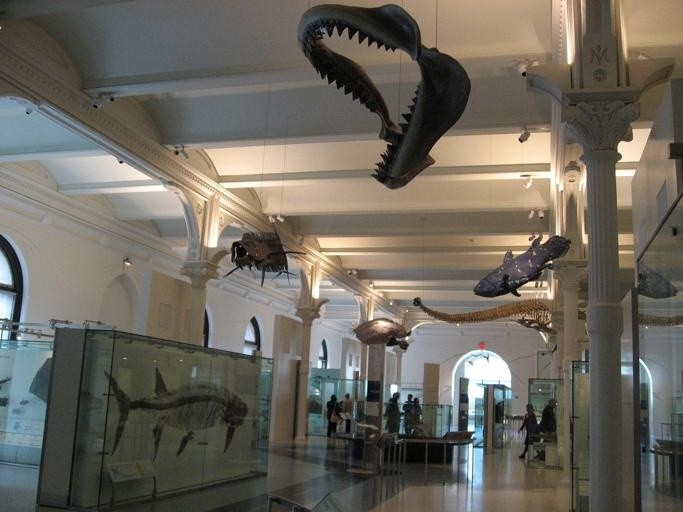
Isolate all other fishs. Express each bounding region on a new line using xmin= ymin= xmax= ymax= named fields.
xmin=103 ymin=367 xmax=248 ymax=464
xmin=356 ymin=423 xmax=379 ymax=432
xmin=473 ymin=234 xmax=571 ymax=297
xmin=356 ymin=318 xmax=409 ymax=346
xmin=637 ymin=259 xmax=678 ymax=299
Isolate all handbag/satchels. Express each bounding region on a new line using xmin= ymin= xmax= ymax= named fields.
xmin=331 ymin=413 xmax=338 ymax=422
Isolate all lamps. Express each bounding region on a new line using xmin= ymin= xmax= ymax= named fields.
xmin=120 ymin=257 xmax=132 ymax=270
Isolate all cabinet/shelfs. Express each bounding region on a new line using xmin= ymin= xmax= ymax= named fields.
xmin=474 ymin=386 xmax=513 ymax=448
xmin=536 ymin=350 xmax=553 ymax=378
xmin=308 ymin=368 xmax=340 ymax=432
xmin=383 ymin=383 xmax=424 ymax=403
xmin=0 ymin=338 xmax=55 ymax=466
xmin=525 ymin=378 xmax=565 ymax=470
xmin=321 ymin=378 xmax=365 ymax=435
xmin=634 ymin=193 xmax=683 ymax=512
xmin=569 ymin=360 xmax=589 ymax=511
xmin=35 ymin=329 xmax=275 ymax=512
xmin=349 ymin=400 xmax=454 ymax=439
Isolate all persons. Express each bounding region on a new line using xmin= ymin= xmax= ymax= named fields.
xmin=518 ymin=405 xmax=540 ymax=458
xmin=538 ymin=399 xmax=556 ymax=460
xmin=384 ymin=393 xmax=422 ymax=435
xmin=327 ymin=393 xmax=353 ymax=438
